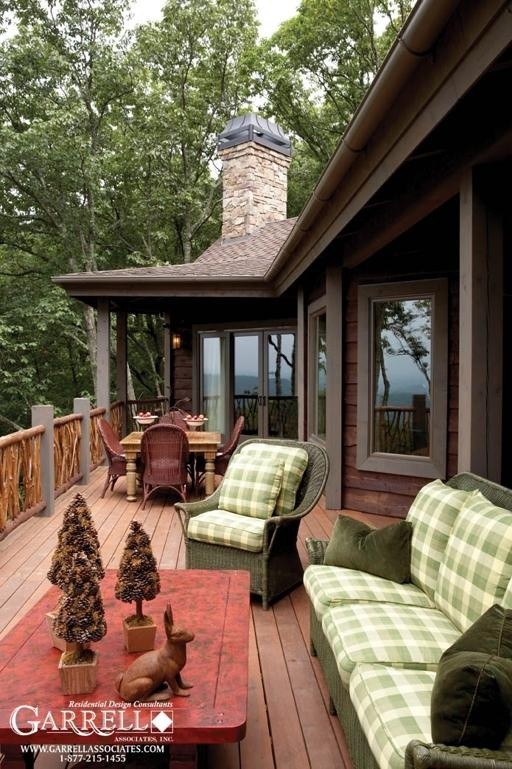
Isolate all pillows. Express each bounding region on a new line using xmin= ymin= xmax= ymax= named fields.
xmin=323 ymin=514 xmax=412 ymax=584
xmin=217 ymin=454 xmax=285 ymax=519
xmin=430 ymin=602 xmax=512 ymax=748
xmin=434 ymin=489 xmax=512 ymax=635
xmin=404 ymin=478 xmax=475 ymax=603
xmin=240 ymin=443 xmax=308 ymax=515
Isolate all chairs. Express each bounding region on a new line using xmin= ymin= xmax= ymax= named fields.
xmin=96 ymin=411 xmax=245 ymax=511
xmin=173 ymin=437 xmax=330 ymax=611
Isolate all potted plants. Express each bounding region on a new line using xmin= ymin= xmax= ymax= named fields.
xmin=45 ymin=491 xmax=160 ymax=696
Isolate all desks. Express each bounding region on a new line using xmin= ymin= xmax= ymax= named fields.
xmin=0 ymin=569 xmax=252 ymax=769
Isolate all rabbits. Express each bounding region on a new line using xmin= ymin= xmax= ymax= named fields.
xmin=113 ymin=600 xmax=196 ymax=702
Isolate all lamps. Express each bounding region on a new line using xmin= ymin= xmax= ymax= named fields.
xmin=171 ymin=331 xmax=184 ymax=351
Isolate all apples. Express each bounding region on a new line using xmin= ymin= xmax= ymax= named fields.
xmin=138 ymin=411 xmax=151 ymax=418
xmin=187 ymin=414 xmax=205 ymax=421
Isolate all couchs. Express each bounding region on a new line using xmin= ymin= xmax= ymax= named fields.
xmin=302 ymin=471 xmax=512 ymax=769
